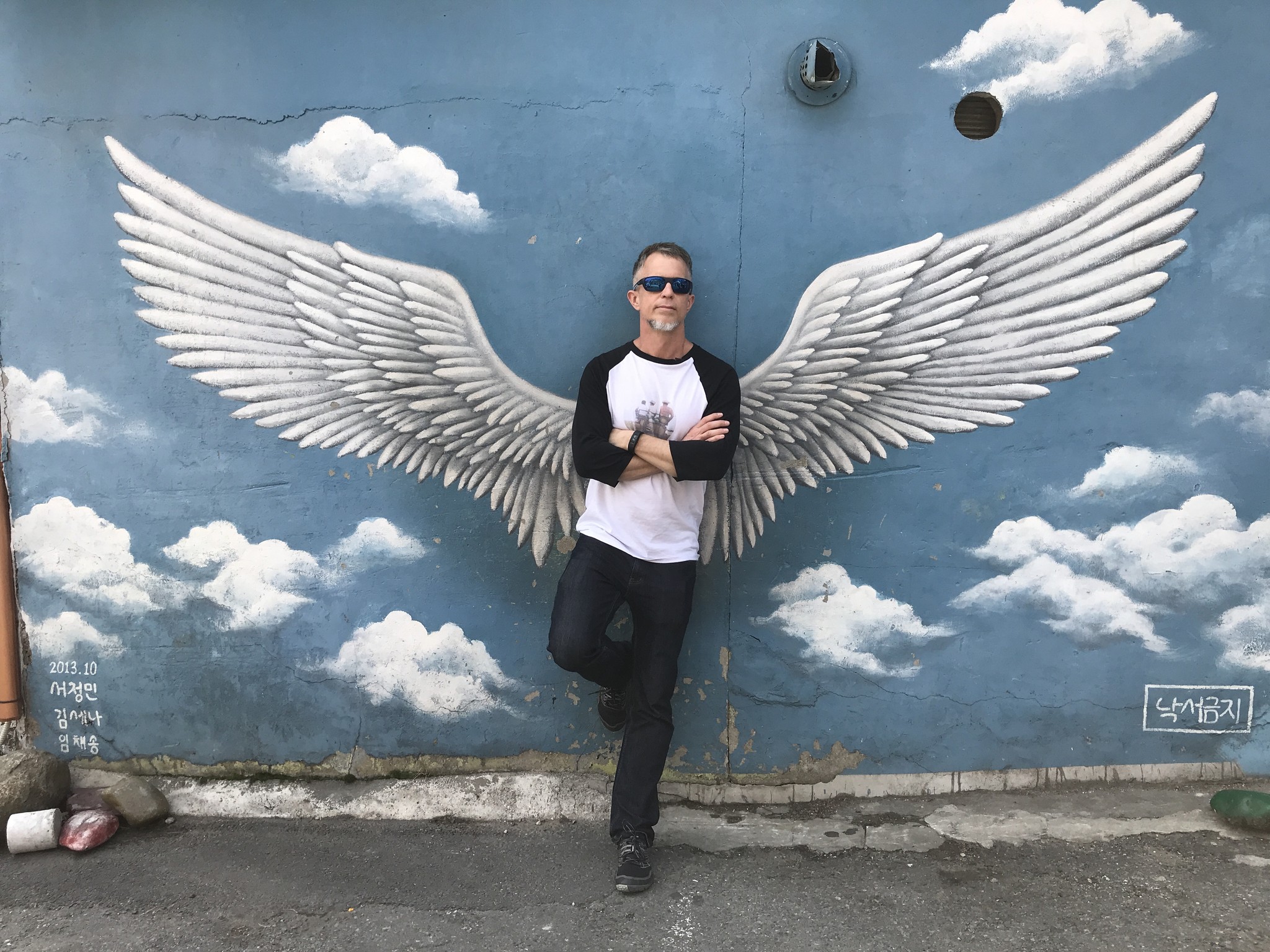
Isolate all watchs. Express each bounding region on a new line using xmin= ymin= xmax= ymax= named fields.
xmin=628 ymin=430 xmax=644 ymax=455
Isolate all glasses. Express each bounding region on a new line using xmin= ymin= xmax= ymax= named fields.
xmin=634 ymin=277 xmax=694 ymax=294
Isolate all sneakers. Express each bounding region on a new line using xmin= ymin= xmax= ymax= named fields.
xmin=615 ymin=821 xmax=654 ymax=892
xmin=588 ymin=680 xmax=628 ymax=732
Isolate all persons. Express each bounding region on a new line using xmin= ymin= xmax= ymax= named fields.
xmin=544 ymin=240 xmax=743 ymax=893
